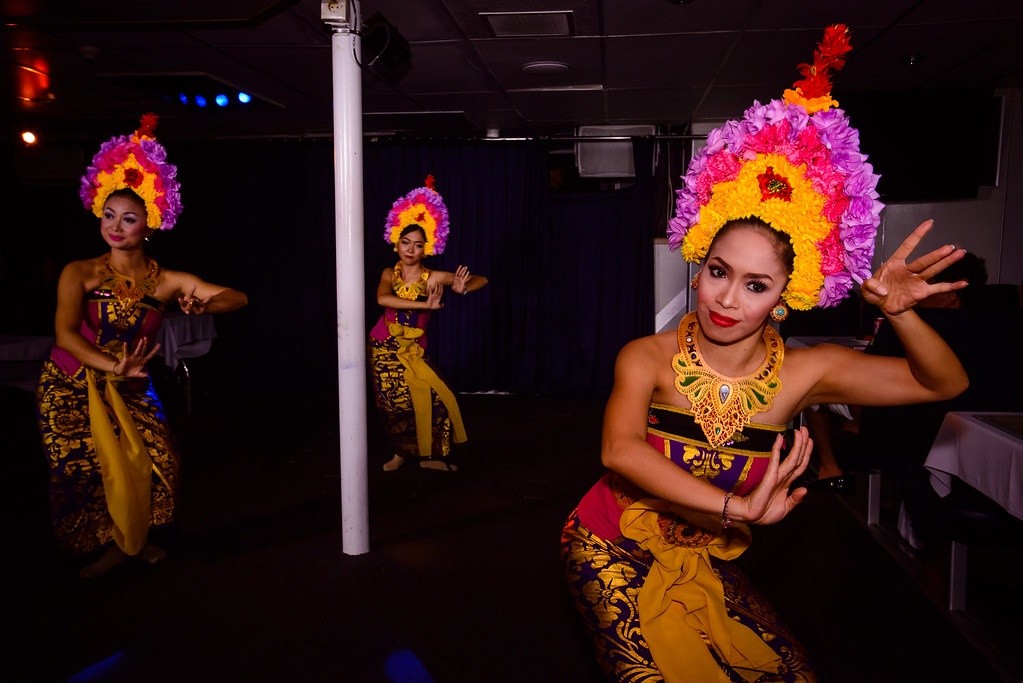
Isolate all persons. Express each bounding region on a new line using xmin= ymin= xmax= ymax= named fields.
xmin=560 ymin=25 xmax=1023 ymax=683
xmin=367 ymin=175 xmax=490 ymax=472
xmin=36 ymin=111 xmax=250 ymax=577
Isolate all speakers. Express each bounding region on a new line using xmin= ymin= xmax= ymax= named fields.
xmin=691 ymin=121 xmax=726 ymax=159
xmin=578 ymin=125 xmax=657 ymax=178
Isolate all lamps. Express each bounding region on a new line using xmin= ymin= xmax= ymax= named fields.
xmin=364 ymin=12 xmax=413 ymax=80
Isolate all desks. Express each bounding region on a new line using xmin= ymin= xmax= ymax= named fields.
xmin=0 ymin=334 xmax=58 ymax=508
xmin=924 ymin=401 xmax=1023 ymax=520
xmin=787 ymin=332 xmax=872 ymax=484
xmin=160 ymin=313 xmax=224 ymax=435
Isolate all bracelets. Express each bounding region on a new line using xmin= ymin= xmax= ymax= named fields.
xmin=463 ymin=285 xmax=468 ymax=294
xmin=720 ymin=491 xmax=734 ymax=529
xmin=112 ymin=362 xmax=119 ymax=372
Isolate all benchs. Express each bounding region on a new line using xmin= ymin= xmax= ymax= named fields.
xmin=847 ymin=282 xmax=1023 ymax=588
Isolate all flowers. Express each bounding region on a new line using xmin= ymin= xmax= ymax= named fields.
xmin=382 ymin=172 xmax=452 ymax=257
xmin=77 ymin=113 xmax=184 ymax=234
xmin=667 ymin=22 xmax=892 ymax=312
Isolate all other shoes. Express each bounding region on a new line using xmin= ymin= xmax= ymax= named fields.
xmin=807 ymin=473 xmax=848 ymax=493
